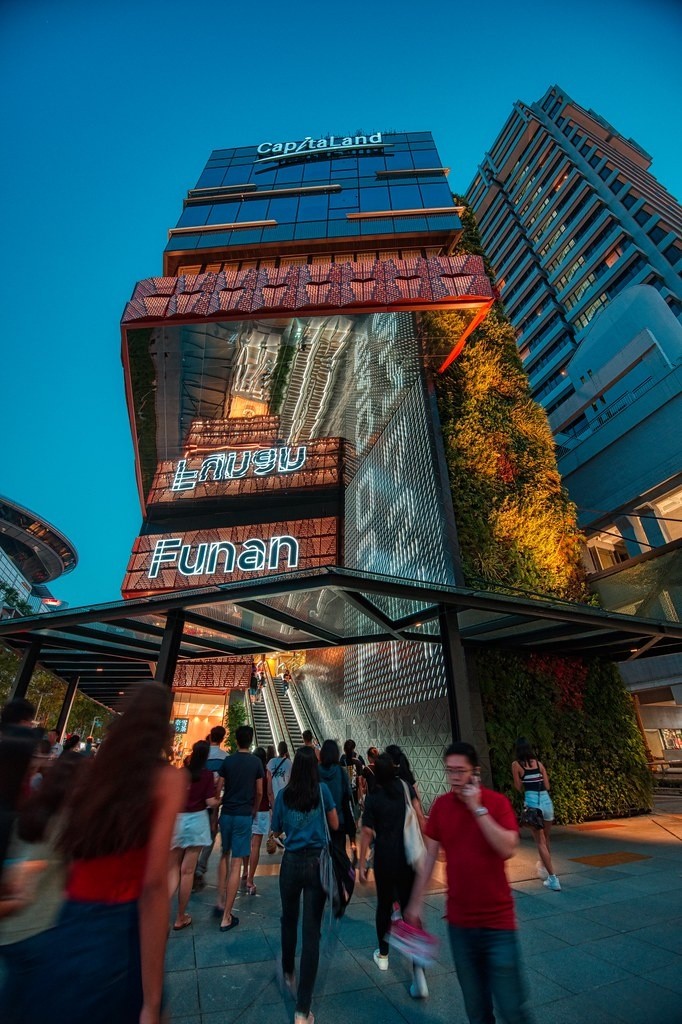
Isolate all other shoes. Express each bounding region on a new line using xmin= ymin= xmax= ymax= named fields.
xmin=193 ymin=870 xmax=206 ymax=892
xmin=240 ymin=874 xmax=256 ymax=894
xmin=283 ymin=973 xmax=296 ymax=987
xmin=294 ymin=1010 xmax=314 ymax=1024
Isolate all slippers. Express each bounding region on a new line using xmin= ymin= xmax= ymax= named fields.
xmin=219 ymin=913 xmax=239 ymax=932
xmin=214 ymin=905 xmax=225 ymax=917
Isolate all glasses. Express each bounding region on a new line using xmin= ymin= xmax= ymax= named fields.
xmin=445 ymin=768 xmax=472 ymax=777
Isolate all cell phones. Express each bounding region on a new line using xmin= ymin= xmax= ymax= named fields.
xmin=468 ymin=772 xmax=482 ymax=787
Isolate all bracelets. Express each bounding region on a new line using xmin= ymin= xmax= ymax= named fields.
xmin=472 ymin=807 xmax=488 ymax=818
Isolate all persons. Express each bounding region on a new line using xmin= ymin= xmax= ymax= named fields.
xmin=0 ymin=669 xmax=562 ymax=1024
xmin=511 ymin=741 xmax=562 ymax=890
xmin=402 ymin=741 xmax=533 ymax=1024
xmin=282 ymin=670 xmax=291 ymax=697
xmin=266 ymin=745 xmax=339 ymax=1024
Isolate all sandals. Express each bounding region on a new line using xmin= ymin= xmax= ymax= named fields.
xmin=174 ymin=913 xmax=191 ymax=930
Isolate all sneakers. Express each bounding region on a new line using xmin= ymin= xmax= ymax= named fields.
xmin=273 ymin=835 xmax=285 ymax=848
xmin=409 ymin=974 xmax=428 ymax=998
xmin=542 ymin=874 xmax=563 ymax=891
xmin=374 ymin=949 xmax=388 ymax=970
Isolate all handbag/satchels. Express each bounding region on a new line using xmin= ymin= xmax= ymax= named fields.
xmin=520 ymin=807 xmax=545 ymax=829
xmin=383 ymin=914 xmax=441 ymax=970
xmin=341 ymin=767 xmax=357 ymax=843
xmin=400 ymin=778 xmax=427 ymax=875
xmin=320 ymin=846 xmax=338 ymax=902
